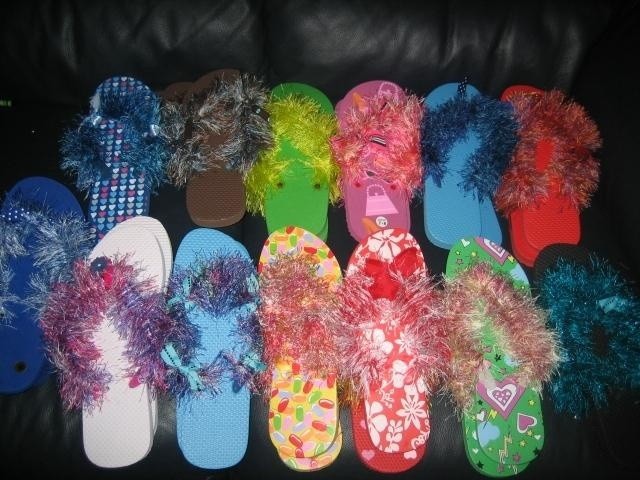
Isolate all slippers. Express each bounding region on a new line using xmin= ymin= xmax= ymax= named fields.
xmin=498 ymin=83 xmax=584 ymax=270
xmin=158 ymin=223 xmax=260 ymax=470
xmin=77 ymin=212 xmax=174 ymax=471
xmin=420 ymin=79 xmax=504 ymax=251
xmin=0 ymin=172 xmax=90 ymax=399
xmin=260 ymin=78 xmax=335 ymax=246
xmin=532 ymin=241 xmax=640 ymax=469
xmin=444 ymin=232 xmax=549 ymax=479
xmin=343 ymin=226 xmax=435 ymax=475
xmin=332 ymin=77 xmax=412 ymax=245
xmin=255 ymin=223 xmax=349 ymax=473
xmin=84 ymin=73 xmax=166 ymax=240
xmin=157 ymin=64 xmax=251 ymax=229
